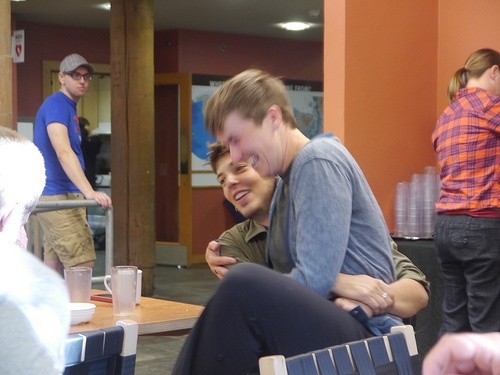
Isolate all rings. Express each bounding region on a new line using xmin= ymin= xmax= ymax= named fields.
xmin=382 ymin=293 xmax=388 ymax=298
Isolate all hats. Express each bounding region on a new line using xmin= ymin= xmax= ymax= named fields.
xmin=60 ymin=53 xmax=95 ymax=72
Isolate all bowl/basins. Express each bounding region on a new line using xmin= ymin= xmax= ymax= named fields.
xmin=70 ymin=302 xmax=96 ymax=325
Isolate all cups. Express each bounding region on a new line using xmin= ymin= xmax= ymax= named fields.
xmin=420 ymin=166 xmax=437 ymax=239
xmin=405 ymin=173 xmax=423 ymax=240
xmin=393 ymin=180 xmax=408 ymax=238
xmin=63 ymin=267 xmax=93 ymax=303
xmin=103 ymin=265 xmax=142 ymax=317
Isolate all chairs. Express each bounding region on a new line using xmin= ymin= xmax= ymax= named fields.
xmin=62 ymin=320 xmax=139 ymax=375
xmin=259 ymin=325 xmax=422 ymax=375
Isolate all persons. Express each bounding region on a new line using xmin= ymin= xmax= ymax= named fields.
xmin=0 ymin=123 xmax=72 ymax=375
xmin=171 ymin=69 xmax=410 ymax=375
xmin=77 ymin=117 xmax=99 ymax=193
xmin=422 ymin=331 xmax=499 ymax=375
xmin=207 ymin=140 xmax=433 ymax=325
xmin=31 ymin=52 xmax=114 ymax=284
xmin=431 ymin=48 xmax=500 ymax=345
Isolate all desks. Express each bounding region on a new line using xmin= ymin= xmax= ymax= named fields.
xmin=68 ymin=289 xmax=205 ymax=336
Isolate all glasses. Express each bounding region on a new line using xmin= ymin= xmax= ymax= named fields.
xmin=64 ymin=71 xmax=93 ymax=80
